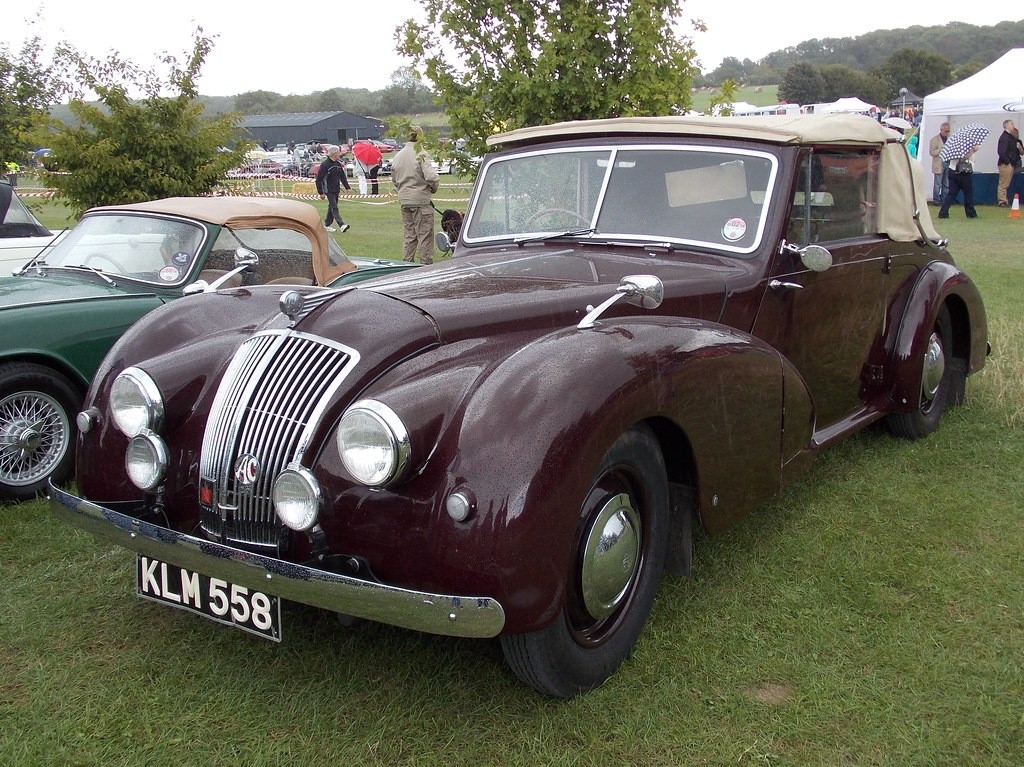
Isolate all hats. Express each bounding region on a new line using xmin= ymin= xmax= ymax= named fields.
xmin=408 ymin=125 xmax=423 ymax=141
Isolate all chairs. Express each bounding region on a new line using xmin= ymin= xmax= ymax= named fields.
xmin=264 ymin=276 xmax=313 ymax=285
xmin=199 ymin=269 xmax=242 ymax=289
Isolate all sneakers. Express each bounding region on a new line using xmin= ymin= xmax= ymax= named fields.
xmin=340 ymin=225 xmax=350 ymax=233
xmin=324 ymin=225 xmax=337 ymax=232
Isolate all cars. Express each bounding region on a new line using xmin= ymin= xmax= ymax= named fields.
xmin=216 ymin=139 xmax=483 ymax=177
xmin=816 ymin=152 xmax=872 ymax=191
xmin=1 ymin=192 xmax=175 ymax=278
xmin=45 ymin=111 xmax=990 ymax=696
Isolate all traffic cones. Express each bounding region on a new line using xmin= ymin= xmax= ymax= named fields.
xmin=1008 ymin=194 xmax=1022 ymax=218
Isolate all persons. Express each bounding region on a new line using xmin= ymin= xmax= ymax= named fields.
xmin=315 ymin=147 xmax=352 ymax=233
xmin=877 ymin=105 xmax=922 ymax=135
xmin=354 ymin=158 xmax=379 ymax=195
xmin=6 ymin=161 xmax=20 ymax=189
xmin=392 ymin=125 xmax=439 ymax=265
xmin=929 ymin=122 xmax=950 ymax=207
xmin=937 ymin=146 xmax=978 ymax=218
xmin=997 ymin=120 xmax=1024 ymax=207
xmin=262 ymin=139 xmax=268 ymax=152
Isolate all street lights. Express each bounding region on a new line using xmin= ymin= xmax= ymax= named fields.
xmin=899 ymin=87 xmax=908 ymax=118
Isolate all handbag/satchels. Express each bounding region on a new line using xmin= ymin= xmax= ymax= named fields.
xmin=954 ymin=158 xmax=973 ymax=175
xmin=365 ymin=172 xmax=370 ymax=179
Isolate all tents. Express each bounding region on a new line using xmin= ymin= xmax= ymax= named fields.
xmin=918 ymin=48 xmax=1024 ymax=206
xmin=888 ymin=89 xmax=924 ymax=113
xmin=823 ymin=98 xmax=873 ymax=112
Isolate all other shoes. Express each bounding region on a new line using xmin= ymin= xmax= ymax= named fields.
xmin=999 ymin=201 xmax=1012 ymax=207
xmin=932 ymin=202 xmax=943 ymax=206
xmin=966 ymin=213 xmax=977 ymax=218
xmin=938 ymin=214 xmax=949 ymax=218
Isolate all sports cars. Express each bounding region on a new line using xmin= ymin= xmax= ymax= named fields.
xmin=0 ymin=196 xmax=423 ymax=496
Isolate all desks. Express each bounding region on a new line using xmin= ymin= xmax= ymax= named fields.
xmin=942 ymin=170 xmax=1024 ymax=205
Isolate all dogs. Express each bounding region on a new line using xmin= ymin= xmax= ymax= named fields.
xmin=440 ymin=207 xmax=468 ymax=259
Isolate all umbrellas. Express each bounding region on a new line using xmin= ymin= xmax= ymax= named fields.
xmin=354 ymin=142 xmax=383 ymax=163
xmin=939 ymin=122 xmax=989 ymax=162
xmin=884 ymin=117 xmax=912 ymax=129
